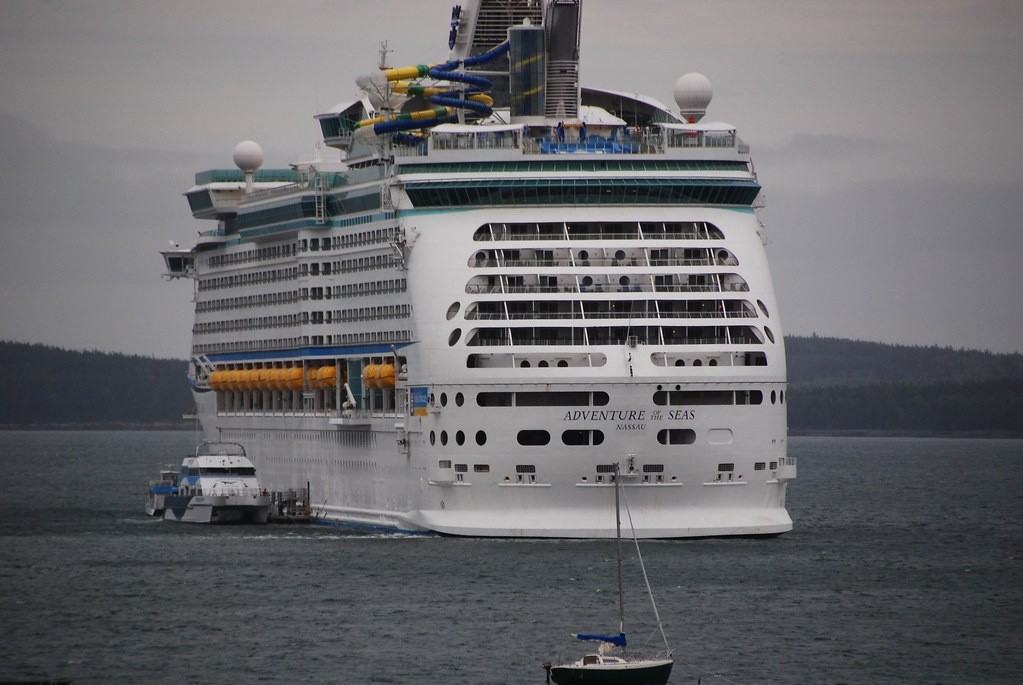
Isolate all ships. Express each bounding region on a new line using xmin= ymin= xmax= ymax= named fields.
xmin=160 ymin=0 xmax=798 ymax=539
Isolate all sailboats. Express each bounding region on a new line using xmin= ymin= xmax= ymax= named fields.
xmin=549 ymin=463 xmax=675 ymax=685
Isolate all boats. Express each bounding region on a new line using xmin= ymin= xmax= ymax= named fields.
xmin=208 ymin=363 xmax=396 ymax=391
xmin=145 ymin=440 xmax=311 ymax=523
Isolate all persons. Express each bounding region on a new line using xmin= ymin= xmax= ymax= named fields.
xmin=579 ymin=122 xmax=587 ymax=143
xmin=552 ymin=122 xmax=565 ymax=143
xmin=633 ymin=125 xmax=641 ymax=140
xmin=524 ymin=126 xmax=531 ymax=136
xmin=262 ymin=489 xmax=268 ymax=496
